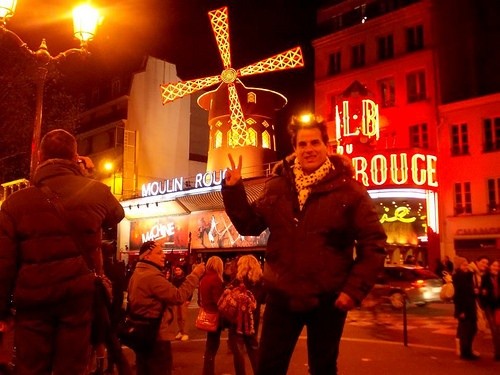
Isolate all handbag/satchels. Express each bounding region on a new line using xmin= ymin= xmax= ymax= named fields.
xmin=115 ymin=310 xmax=160 ymax=353
xmin=95 ymin=274 xmax=113 ymax=323
xmin=194 ymin=307 xmax=220 ymax=333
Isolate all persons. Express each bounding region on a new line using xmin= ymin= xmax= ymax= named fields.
xmin=0 ymin=129 xmax=125 ymax=375
xmin=125 ymin=241 xmax=263 ymax=375
xmin=403 ymin=254 xmax=500 ymax=375
xmin=221 ymin=111 xmax=388 ymax=375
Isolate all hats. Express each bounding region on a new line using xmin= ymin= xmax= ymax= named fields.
xmin=139 ymin=240 xmax=159 ymax=260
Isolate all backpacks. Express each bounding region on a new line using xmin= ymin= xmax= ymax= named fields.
xmin=218 ymin=285 xmax=258 ymax=336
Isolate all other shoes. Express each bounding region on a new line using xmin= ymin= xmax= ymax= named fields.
xmin=463 ymin=354 xmax=478 ymax=360
